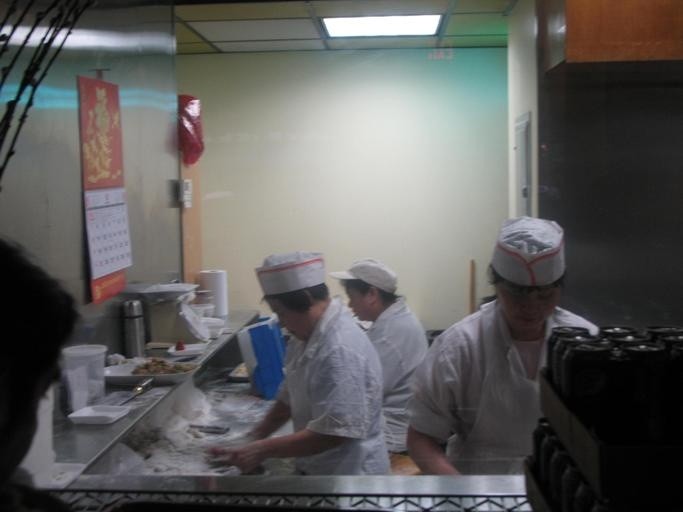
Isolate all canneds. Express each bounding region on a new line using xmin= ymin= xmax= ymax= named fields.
xmin=532 ymin=324 xmax=683 ymax=512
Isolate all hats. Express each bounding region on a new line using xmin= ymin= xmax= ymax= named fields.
xmin=329 ymin=258 xmax=397 ymax=294
xmin=255 ymin=251 xmax=326 ymax=296
xmin=494 ymin=216 xmax=567 ymax=287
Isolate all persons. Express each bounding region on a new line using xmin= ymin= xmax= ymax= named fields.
xmin=405 ymin=216 xmax=598 ymax=474
xmin=329 ymin=257 xmax=430 ymax=475
xmin=203 ymin=249 xmax=392 ymax=476
xmin=0 ymin=234 xmax=81 ymax=511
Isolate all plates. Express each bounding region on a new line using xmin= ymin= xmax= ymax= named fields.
xmin=102 ymin=355 xmax=201 ymax=385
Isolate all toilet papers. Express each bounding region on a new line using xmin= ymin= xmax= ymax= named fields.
xmin=199 ymin=270 xmax=228 ymax=318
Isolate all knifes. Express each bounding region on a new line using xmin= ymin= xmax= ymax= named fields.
xmin=115 ymin=375 xmax=156 ymax=405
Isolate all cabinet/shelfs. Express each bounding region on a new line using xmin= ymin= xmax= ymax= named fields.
xmin=540 ymin=0 xmax=683 ymax=76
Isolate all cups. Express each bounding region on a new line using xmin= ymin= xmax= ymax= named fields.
xmin=122 ymin=299 xmax=147 ymax=359
xmin=59 ymin=342 xmax=107 ymax=412
